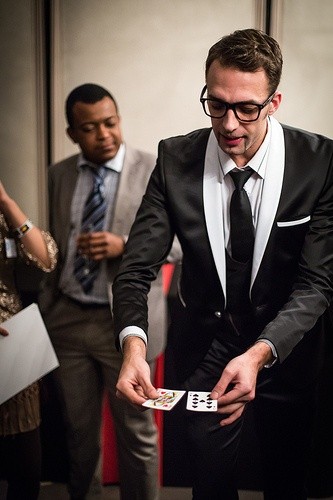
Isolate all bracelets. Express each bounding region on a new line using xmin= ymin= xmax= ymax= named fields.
xmin=15 ymin=220 xmax=32 ymax=238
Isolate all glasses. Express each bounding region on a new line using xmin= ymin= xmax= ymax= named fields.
xmin=199 ymin=85 xmax=275 ymax=122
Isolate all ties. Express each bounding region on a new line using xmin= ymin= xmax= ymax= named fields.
xmin=226 ymin=166 xmax=253 ymax=329
xmin=74 ymin=166 xmax=106 ymax=295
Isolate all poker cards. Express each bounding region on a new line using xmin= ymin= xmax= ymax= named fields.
xmin=186 ymin=391 xmax=218 ymax=412
xmin=141 ymin=388 xmax=186 ymax=411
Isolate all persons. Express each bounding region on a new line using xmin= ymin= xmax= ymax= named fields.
xmin=0 ymin=180 xmax=53 ymax=500
xmin=111 ymin=29 xmax=333 ymax=500
xmin=42 ymin=83 xmax=184 ymax=500
